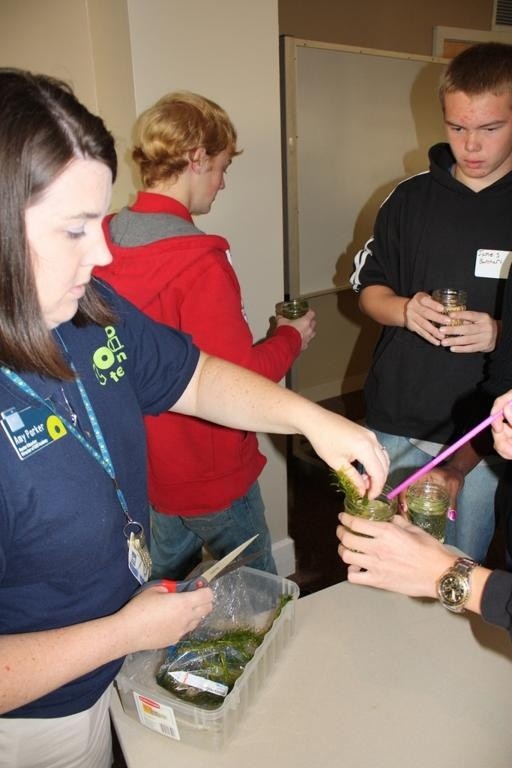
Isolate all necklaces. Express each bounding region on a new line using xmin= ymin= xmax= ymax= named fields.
xmin=51 ymin=380 xmax=79 ymax=428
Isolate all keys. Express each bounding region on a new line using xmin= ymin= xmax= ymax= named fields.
xmin=133 ymin=529 xmax=152 ymax=569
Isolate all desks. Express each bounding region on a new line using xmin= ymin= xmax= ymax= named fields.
xmin=108 ymin=544 xmax=512 ymax=768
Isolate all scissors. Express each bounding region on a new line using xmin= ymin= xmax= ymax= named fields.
xmin=129 ymin=533 xmax=259 ymax=600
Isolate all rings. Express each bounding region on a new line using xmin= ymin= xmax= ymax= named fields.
xmin=201 ymin=616 xmax=205 ymax=622
xmin=379 ymin=446 xmax=386 ymax=451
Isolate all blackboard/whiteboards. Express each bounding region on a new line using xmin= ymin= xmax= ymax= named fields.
xmin=284 ymin=36 xmax=454 ymax=301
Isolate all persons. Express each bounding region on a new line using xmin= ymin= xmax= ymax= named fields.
xmin=334 ymin=385 xmax=510 ymax=629
xmin=86 ymin=86 xmax=320 ymax=631
xmin=396 ymin=412 xmax=494 ymax=524
xmin=344 ymin=40 xmax=510 ymax=582
xmin=2 ymin=63 xmax=393 ymax=767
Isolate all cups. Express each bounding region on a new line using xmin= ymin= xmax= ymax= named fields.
xmin=432 ymin=289 xmax=466 ymax=347
xmin=344 ymin=483 xmax=398 ymax=552
xmin=406 ymin=482 xmax=450 ymax=545
xmin=279 ymin=299 xmax=307 ymax=319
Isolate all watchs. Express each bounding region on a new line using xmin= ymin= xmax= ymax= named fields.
xmin=435 ymin=556 xmax=483 ymax=616
